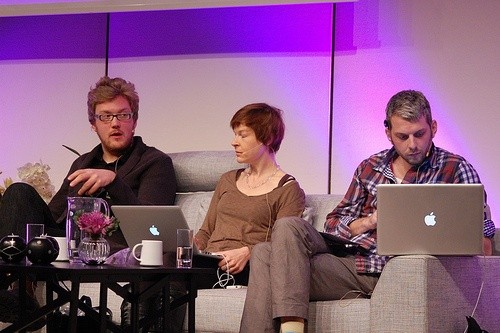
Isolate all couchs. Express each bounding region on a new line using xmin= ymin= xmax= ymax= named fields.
xmin=0 ymin=150 xmax=500 ymax=333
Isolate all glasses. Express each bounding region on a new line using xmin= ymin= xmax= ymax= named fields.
xmin=95 ymin=112 xmax=134 ymax=121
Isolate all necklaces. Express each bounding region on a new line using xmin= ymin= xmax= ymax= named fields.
xmin=246 ymin=164 xmax=280 ymax=188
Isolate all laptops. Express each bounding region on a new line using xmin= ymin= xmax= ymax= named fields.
xmin=111 ymin=204 xmax=224 ymax=259
xmin=376 ymin=183 xmax=484 ymax=256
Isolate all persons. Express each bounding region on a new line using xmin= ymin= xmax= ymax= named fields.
xmin=120 ymin=102 xmax=305 ymax=333
xmin=239 ymin=90 xmax=496 ymax=333
xmin=0 ymin=75 xmax=177 ymax=333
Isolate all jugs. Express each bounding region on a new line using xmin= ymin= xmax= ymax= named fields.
xmin=66 ymin=197 xmax=109 ymax=264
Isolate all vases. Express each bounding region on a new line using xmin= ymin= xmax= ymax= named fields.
xmin=78 ymin=233 xmax=111 ymax=265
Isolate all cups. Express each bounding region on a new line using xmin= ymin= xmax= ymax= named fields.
xmin=27 ymin=224 xmax=44 ymax=263
xmin=176 ymin=229 xmax=194 ymax=268
xmin=133 ymin=240 xmax=163 ymax=266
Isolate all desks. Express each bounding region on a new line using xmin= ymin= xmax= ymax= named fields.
xmin=0 ymin=259 xmax=222 ymax=333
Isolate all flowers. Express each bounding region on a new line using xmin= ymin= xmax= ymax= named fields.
xmin=76 ymin=211 xmax=120 ymax=241
xmin=17 ymin=162 xmax=56 ymax=202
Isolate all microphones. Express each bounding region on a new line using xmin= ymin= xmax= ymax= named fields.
xmin=426 ymin=141 xmax=432 ymax=157
